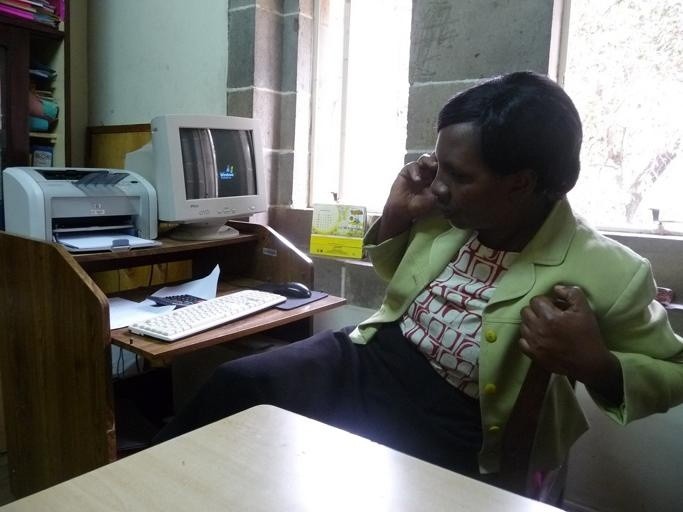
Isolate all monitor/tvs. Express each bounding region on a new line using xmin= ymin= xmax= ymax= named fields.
xmin=121 ymin=113 xmax=269 ymax=242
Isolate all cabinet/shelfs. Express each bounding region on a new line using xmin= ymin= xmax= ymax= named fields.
xmin=0 ymin=0 xmax=71 ymax=169
xmin=0 ymin=216 xmax=349 ymax=500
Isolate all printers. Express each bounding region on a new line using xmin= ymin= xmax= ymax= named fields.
xmin=2 ymin=167 xmax=163 ymax=255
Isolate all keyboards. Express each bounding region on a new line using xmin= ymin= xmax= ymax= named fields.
xmin=127 ymin=289 xmax=288 ymax=342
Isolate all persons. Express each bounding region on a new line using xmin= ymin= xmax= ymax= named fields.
xmin=148 ymin=67 xmax=683 ymax=499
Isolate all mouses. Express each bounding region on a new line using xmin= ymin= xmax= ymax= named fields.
xmin=275 ymin=280 xmax=313 ymax=300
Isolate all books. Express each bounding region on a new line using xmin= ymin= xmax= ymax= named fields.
xmin=0 ymin=0 xmax=61 ymax=27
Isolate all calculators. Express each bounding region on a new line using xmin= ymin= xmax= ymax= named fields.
xmin=146 ymin=294 xmax=206 ymax=309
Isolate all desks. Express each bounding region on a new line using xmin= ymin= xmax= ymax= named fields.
xmin=0 ymin=403 xmax=572 ymax=512
